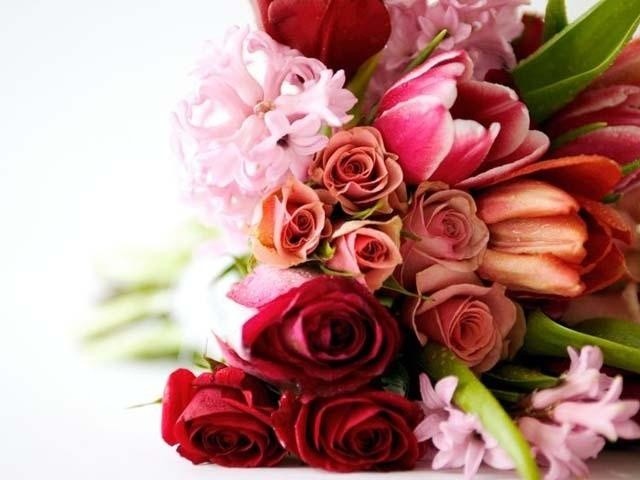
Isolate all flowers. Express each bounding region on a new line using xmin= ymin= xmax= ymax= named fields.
xmin=162 ymin=0 xmax=638 ymax=480
